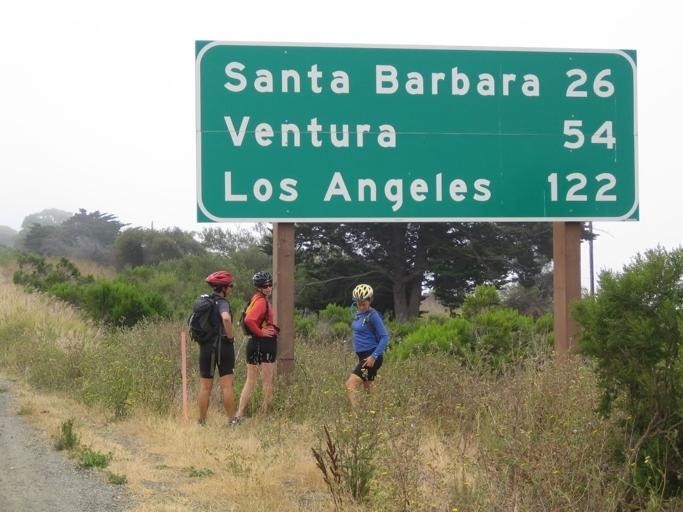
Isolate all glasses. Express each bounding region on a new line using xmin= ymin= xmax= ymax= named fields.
xmin=260 ymin=284 xmax=272 ymax=289
xmin=227 ymin=283 xmax=233 ymax=287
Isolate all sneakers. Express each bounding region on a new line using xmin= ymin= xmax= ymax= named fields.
xmin=232 ymin=416 xmax=241 ymax=423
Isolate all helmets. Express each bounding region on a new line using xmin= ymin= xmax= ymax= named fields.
xmin=352 ymin=284 xmax=372 ymax=301
xmin=205 ymin=271 xmax=232 ymax=287
xmin=253 ymin=272 xmax=272 ymax=286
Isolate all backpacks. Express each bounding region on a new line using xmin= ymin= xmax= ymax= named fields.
xmin=189 ymin=294 xmax=224 ymax=345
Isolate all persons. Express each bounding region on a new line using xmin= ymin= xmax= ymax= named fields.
xmin=193 ymin=270 xmax=243 ymax=427
xmin=343 ymin=283 xmax=390 ymax=413
xmin=232 ymin=269 xmax=280 ymax=426
